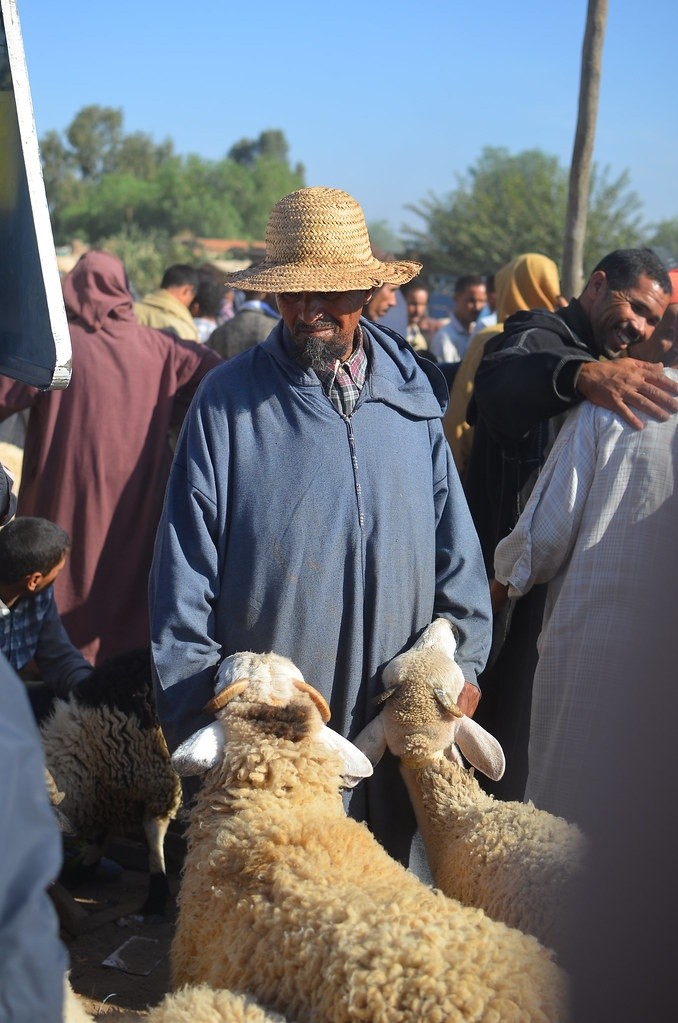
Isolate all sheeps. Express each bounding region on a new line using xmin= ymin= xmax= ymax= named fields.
xmin=161 ymin=649 xmax=575 ymax=1023
xmin=35 ymin=689 xmax=182 ymax=918
xmin=93 ymin=982 xmax=290 ymax=1023
xmin=351 ymin=618 xmax=678 ymax=1023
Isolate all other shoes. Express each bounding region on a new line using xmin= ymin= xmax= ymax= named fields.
xmin=63 ymin=847 xmax=123 ymax=882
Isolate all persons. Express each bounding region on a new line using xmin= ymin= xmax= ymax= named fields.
xmin=0 ymin=187 xmax=678 ymax=1022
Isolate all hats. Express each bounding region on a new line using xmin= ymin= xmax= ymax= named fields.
xmin=223 ymin=190 xmax=423 ymax=293
